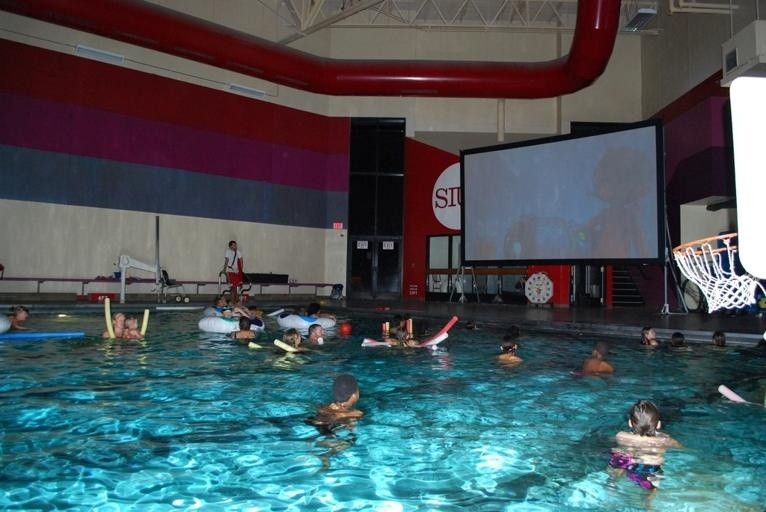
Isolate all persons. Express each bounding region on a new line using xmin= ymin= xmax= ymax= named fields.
xmin=102 ymin=312 xmax=125 ymax=339
xmin=121 ymin=314 xmax=144 ymax=341
xmin=309 ymin=374 xmax=364 ymax=469
xmin=710 ymin=331 xmax=728 ymax=352
xmin=576 ymin=338 xmax=614 ymax=375
xmin=210 ymin=295 xmax=336 ymax=371
xmin=495 ymin=334 xmax=523 ymax=366
xmin=640 ymin=326 xmax=659 ymax=348
xmin=666 ymin=332 xmax=690 ymax=352
xmin=373 ymin=313 xmax=433 ymax=350
xmin=505 ymin=324 xmax=529 ymax=342
xmin=7 ymin=307 xmax=34 ymax=331
xmin=606 ymin=398 xmax=683 ymax=510
xmin=222 ymin=240 xmax=243 ymax=301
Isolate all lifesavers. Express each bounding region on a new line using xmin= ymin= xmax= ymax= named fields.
xmin=198 ymin=307 xmax=337 ymax=333
xmin=0 ymin=314 xmax=10 ymax=333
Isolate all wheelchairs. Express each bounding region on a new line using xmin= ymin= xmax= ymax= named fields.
xmin=158 ymin=268 xmax=191 ymax=304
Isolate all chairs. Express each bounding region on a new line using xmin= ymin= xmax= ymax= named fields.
xmin=161 ymin=269 xmax=186 ymax=303
